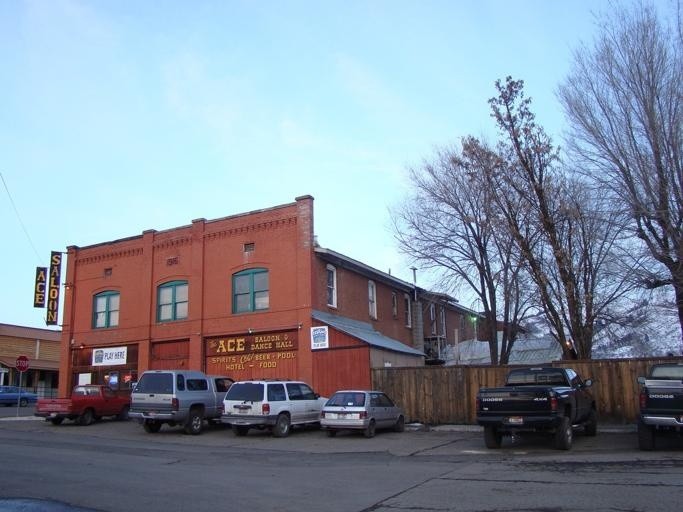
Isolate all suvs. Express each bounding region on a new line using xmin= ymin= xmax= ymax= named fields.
xmin=636 ymin=362 xmax=683 ymax=451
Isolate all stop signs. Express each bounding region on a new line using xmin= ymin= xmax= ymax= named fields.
xmin=15 ymin=355 xmax=30 ymax=372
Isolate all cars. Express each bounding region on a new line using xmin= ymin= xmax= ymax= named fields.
xmin=128 ymin=370 xmax=405 ymax=438
xmin=0 ymin=385 xmax=39 ymax=407
xmin=34 ymin=383 xmax=131 ymax=426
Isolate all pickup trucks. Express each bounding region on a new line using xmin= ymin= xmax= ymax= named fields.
xmin=475 ymin=368 xmax=598 ymax=450
